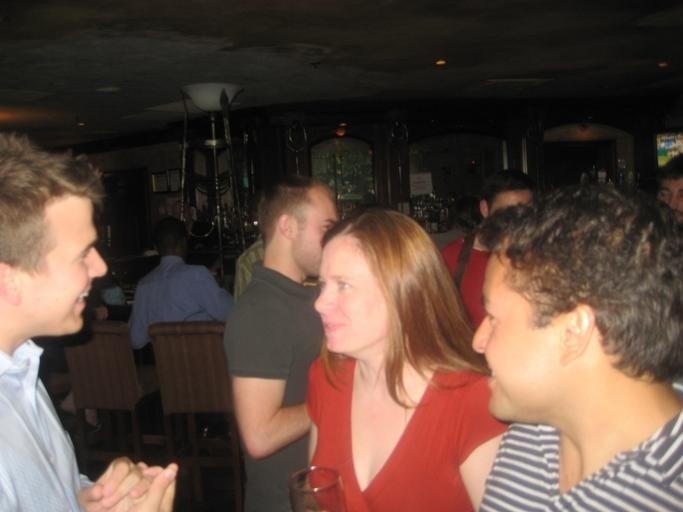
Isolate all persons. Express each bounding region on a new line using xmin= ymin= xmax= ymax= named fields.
xmin=0 ymin=134 xmax=180 ymax=511
xmin=40 ymin=155 xmax=683 ymax=512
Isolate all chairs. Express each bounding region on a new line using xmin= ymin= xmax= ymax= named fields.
xmin=148 ymin=321 xmax=243 ymax=512
xmin=66 ymin=321 xmax=159 ymax=473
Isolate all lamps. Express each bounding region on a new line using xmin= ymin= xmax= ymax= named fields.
xmin=182 ymin=84 xmax=244 ymax=286
